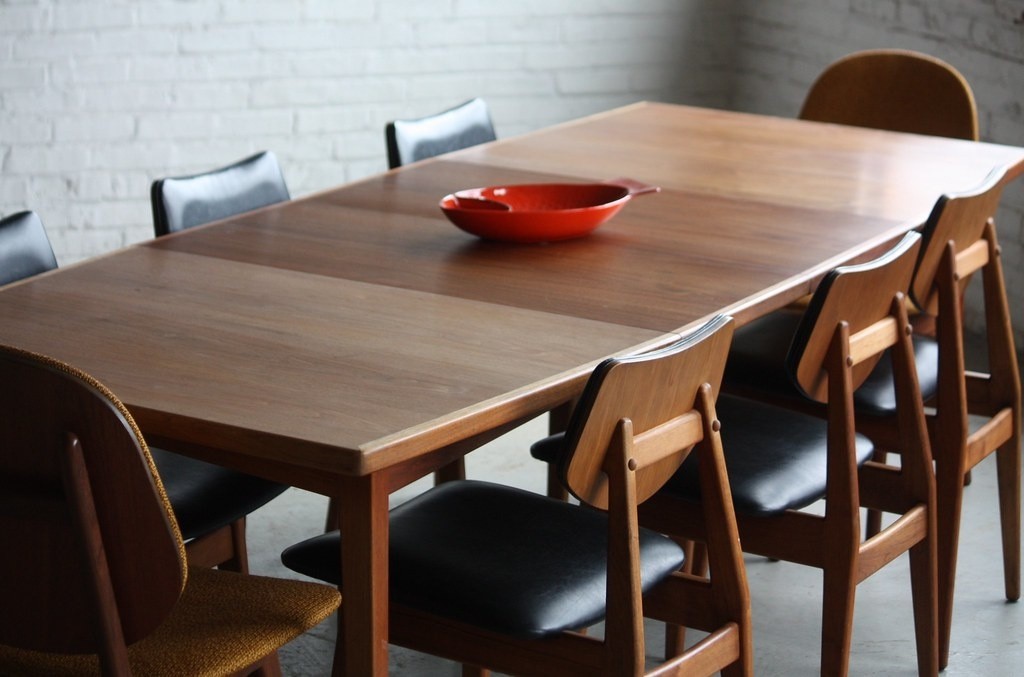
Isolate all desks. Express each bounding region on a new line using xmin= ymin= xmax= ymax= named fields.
xmin=0 ymin=99 xmax=1024 ymax=677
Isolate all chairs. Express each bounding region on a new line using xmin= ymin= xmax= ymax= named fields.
xmin=281 ymin=314 xmax=753 ymax=677
xmin=0 ymin=210 xmax=291 ymax=573
xmin=782 ymin=51 xmax=972 ymax=487
xmin=720 ymin=161 xmax=1024 ymax=671
xmin=384 ymin=96 xmax=571 ymax=503
xmin=530 ymin=229 xmax=938 ymax=677
xmin=150 ymin=147 xmax=466 ymax=534
xmin=0 ymin=345 xmax=342 ymax=677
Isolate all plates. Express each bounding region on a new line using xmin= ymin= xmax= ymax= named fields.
xmin=438 ymin=175 xmax=660 ymax=244
